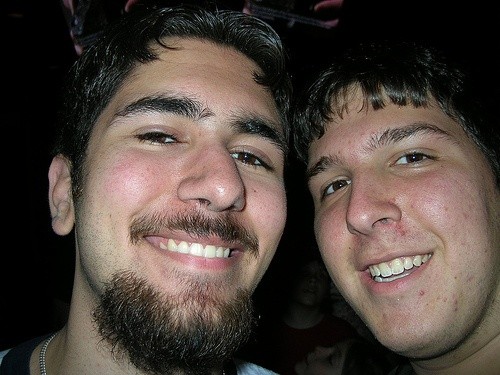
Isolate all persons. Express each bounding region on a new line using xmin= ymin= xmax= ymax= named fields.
xmin=65 ymin=0 xmax=411 ymax=375
xmin=0 ymin=6 xmax=295 ymax=375
xmin=296 ymin=26 xmax=500 ymax=375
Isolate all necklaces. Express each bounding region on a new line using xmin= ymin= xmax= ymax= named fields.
xmin=39 ymin=332 xmax=58 ymax=375
